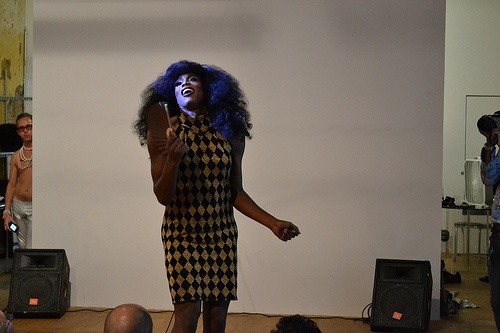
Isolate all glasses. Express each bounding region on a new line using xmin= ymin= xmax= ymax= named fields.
xmin=17 ymin=124 xmax=32 ymax=132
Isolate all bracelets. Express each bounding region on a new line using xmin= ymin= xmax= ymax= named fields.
xmin=3 ymin=209 xmax=11 ymax=219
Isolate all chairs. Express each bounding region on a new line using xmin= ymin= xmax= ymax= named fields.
xmin=453 ymin=208 xmax=492 ymax=264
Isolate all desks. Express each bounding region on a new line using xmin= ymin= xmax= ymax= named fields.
xmin=441 ymin=201 xmax=492 ymax=258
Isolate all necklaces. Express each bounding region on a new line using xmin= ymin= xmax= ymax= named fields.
xmin=17 ymin=143 xmax=32 ymax=170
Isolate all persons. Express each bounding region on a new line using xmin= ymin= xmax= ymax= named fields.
xmin=476 ymin=110 xmax=500 ymax=333
xmin=1 ymin=112 xmax=32 ymax=249
xmin=136 ymin=59 xmax=302 ymax=333
xmin=104 ymin=303 xmax=154 ymax=333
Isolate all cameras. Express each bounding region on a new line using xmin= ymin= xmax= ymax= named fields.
xmin=9 ymin=222 xmax=18 ymax=232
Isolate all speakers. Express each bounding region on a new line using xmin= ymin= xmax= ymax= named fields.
xmin=371 ymin=258 xmax=433 ymax=333
xmin=8 ymin=249 xmax=71 ymax=318
